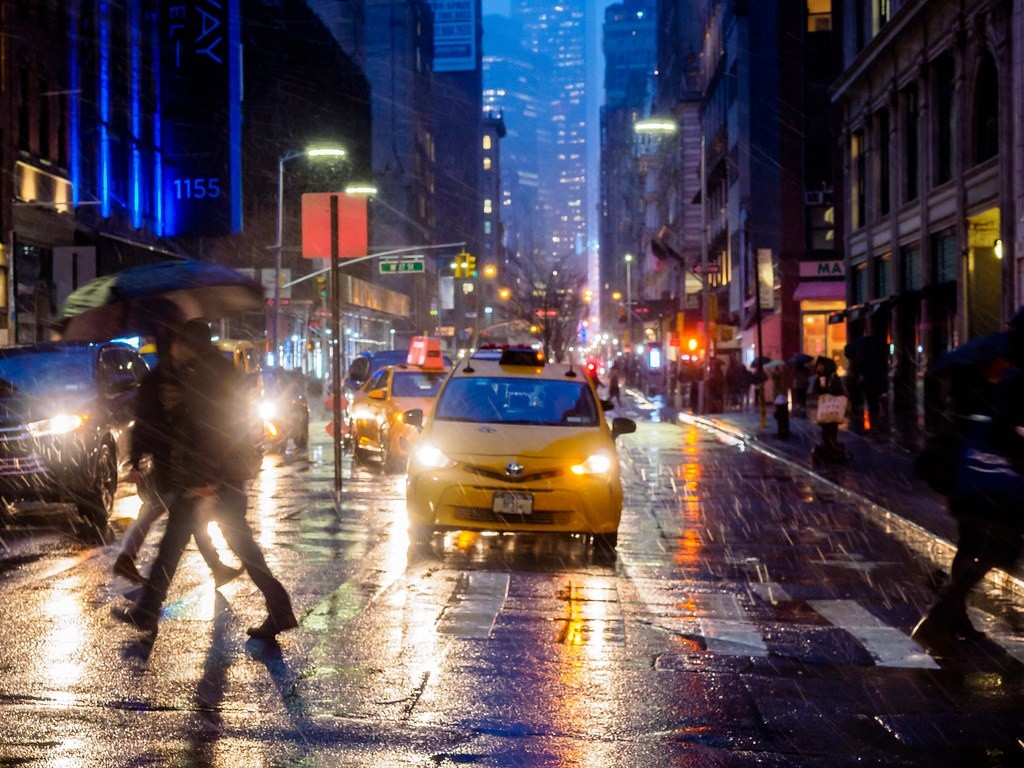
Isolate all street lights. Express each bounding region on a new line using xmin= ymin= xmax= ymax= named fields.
xmin=319 ymin=187 xmax=378 ymax=492
xmin=273 ymin=150 xmax=346 ymax=365
xmin=624 ymin=252 xmax=634 ymax=387
xmin=636 ymin=119 xmax=708 ymax=414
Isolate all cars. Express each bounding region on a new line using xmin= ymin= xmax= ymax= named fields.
xmin=139 ymin=340 xmax=264 ymax=411
xmin=402 ymin=345 xmax=636 ymax=567
xmin=260 ymin=387 xmax=309 ymax=453
xmin=345 ymin=336 xmax=455 ymax=466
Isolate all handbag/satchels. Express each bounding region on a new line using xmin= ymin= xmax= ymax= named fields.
xmin=816 ymin=393 xmax=848 ymax=423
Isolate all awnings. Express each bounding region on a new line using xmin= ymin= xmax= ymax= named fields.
xmin=829 ymin=295 xmax=897 ymax=324
xmin=792 ymin=281 xmax=848 ymax=302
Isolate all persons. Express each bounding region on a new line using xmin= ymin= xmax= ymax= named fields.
xmin=588 ymin=361 xmax=624 ymax=408
xmin=793 ymin=347 xmax=878 ymax=455
xmin=621 ymin=352 xmax=782 ymax=406
xmin=111 ymin=317 xmax=298 ymax=640
xmin=911 ymin=305 xmax=1024 ymax=654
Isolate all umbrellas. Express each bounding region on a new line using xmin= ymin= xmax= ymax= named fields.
xmin=54 ymin=260 xmax=265 ymax=338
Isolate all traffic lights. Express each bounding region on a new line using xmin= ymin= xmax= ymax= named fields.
xmin=467 ymin=256 xmax=477 ymax=279
xmin=317 ymin=277 xmax=328 ymax=301
xmin=452 ymin=254 xmax=463 ymax=279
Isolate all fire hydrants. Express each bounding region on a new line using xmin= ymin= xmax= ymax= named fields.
xmin=773 ymin=393 xmax=792 ymax=440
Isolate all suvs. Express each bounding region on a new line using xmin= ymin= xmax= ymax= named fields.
xmin=1 ymin=340 xmax=151 ymax=528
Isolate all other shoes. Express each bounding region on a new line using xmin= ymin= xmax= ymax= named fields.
xmin=911 ymin=617 xmax=960 ymax=656
xmin=112 ymin=606 xmax=159 ymax=629
xmin=112 ymin=552 xmax=144 ymax=581
xmin=246 ymin=616 xmax=280 ymax=638
xmin=958 ymin=617 xmax=986 ymax=640
xmin=211 ymin=562 xmax=246 ymax=587
